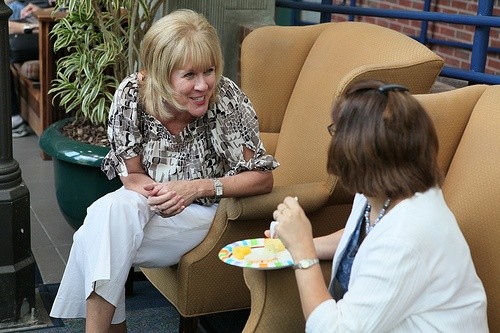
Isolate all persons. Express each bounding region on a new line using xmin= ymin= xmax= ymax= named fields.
xmin=50 ymin=9 xmax=280 ymax=333
xmin=5 ymin=0 xmax=58 ymax=137
xmin=264 ymin=80 xmax=491 ymax=332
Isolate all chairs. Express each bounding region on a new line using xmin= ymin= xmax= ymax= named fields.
xmin=138 ymin=14 xmax=444 ymax=333
xmin=240 ymin=83 xmax=500 ymax=333
xmin=18 ymin=9 xmax=130 ymax=159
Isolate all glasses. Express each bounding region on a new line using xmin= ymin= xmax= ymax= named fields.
xmin=326 ymin=122 xmax=336 ymax=136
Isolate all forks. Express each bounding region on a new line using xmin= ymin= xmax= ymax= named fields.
xmin=269 ymin=195 xmax=298 ymax=239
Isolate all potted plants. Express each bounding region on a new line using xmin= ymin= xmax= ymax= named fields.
xmin=38 ymin=0 xmax=165 ymax=235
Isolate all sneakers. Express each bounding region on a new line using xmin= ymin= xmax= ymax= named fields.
xmin=12 ymin=121 xmax=28 ymax=137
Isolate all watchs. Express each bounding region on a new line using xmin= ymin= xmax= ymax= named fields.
xmin=211 ymin=178 xmax=223 ymax=198
xmin=294 ymin=258 xmax=319 ymax=269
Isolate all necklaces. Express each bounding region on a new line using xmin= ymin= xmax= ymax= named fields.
xmin=364 ymin=198 xmax=390 ymax=234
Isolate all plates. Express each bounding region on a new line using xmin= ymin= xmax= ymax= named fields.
xmin=218 ymin=238 xmax=295 ymax=271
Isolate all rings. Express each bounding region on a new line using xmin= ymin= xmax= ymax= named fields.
xmin=154 ymin=206 xmax=160 ymax=214
